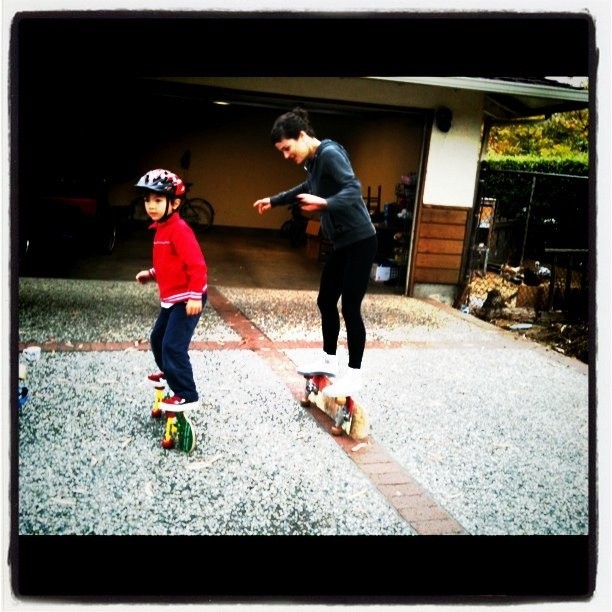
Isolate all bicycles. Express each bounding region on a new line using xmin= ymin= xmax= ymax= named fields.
xmin=128 ymin=182 xmax=213 ymax=231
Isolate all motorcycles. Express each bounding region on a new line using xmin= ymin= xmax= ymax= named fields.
xmin=97 ymin=174 xmax=139 ymax=256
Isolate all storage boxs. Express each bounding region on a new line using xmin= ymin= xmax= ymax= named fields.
xmin=370 ymin=263 xmax=390 ymax=282
xmin=305 ymin=220 xmax=322 ymax=261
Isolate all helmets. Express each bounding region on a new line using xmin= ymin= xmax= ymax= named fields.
xmin=135 ymin=168 xmax=185 ymax=196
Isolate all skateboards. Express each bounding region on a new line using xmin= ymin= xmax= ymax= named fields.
xmin=300 ymin=372 xmax=369 ymax=440
xmin=151 ymin=378 xmax=195 ymax=452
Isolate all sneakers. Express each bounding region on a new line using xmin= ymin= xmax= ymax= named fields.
xmin=297 ymin=356 xmax=336 ymax=378
xmin=321 ymin=373 xmax=363 ymax=397
xmin=147 ymin=370 xmax=169 ymax=387
xmin=159 ymin=394 xmax=198 ymax=413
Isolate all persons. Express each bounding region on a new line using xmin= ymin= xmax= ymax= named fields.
xmin=250 ymin=107 xmax=381 ymax=398
xmin=134 ymin=170 xmax=210 ymax=413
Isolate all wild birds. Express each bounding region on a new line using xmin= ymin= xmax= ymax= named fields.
xmin=534 ymin=260 xmax=551 ymax=276
xmin=481 ymin=288 xmax=519 ymax=319
